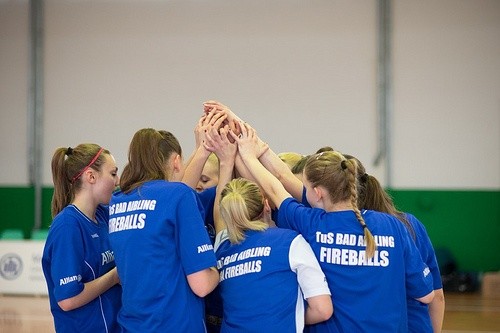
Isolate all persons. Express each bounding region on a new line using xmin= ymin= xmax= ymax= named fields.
xmin=202 ymin=127 xmax=334 ymax=333
xmin=109 ymin=106 xmax=220 ymax=332
xmin=228 ymin=120 xmax=435 ymax=333
xmin=206 ymin=101 xmax=445 ymax=333
xmin=195 ymin=144 xmax=344 ymax=214
xmin=39 ymin=117 xmax=209 ymax=332
